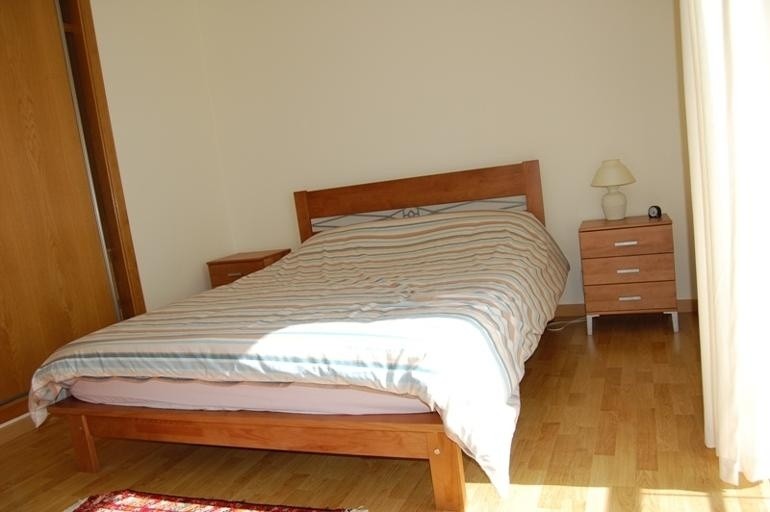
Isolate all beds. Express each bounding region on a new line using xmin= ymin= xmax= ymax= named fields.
xmin=50 ymin=159 xmax=552 ymax=512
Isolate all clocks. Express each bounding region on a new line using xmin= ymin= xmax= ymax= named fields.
xmin=647 ymin=206 xmax=661 ymax=218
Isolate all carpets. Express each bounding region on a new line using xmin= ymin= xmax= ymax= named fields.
xmin=61 ymin=488 xmax=369 ymax=512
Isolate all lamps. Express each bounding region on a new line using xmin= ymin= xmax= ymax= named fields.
xmin=589 ymin=158 xmax=636 ymax=221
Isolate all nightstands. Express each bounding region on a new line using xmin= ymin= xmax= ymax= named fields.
xmin=578 ymin=212 xmax=680 ymax=337
xmin=206 ymin=248 xmax=292 ymax=288
